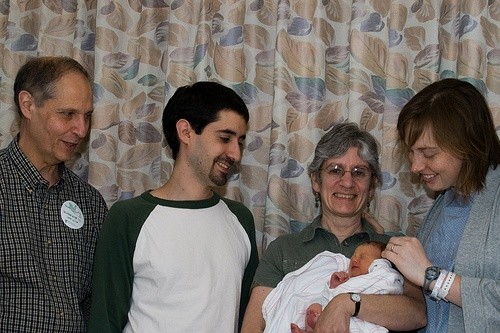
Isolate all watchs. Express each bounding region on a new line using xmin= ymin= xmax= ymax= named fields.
xmin=424 ymin=266 xmax=441 ymax=292
xmin=348 ymin=292 xmax=360 ymax=318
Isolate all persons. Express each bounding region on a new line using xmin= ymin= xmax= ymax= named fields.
xmin=291 ymin=239 xmax=382 ymax=333
xmin=240 ymin=123 xmax=427 ymax=333
xmin=0 ymin=56 xmax=109 ymax=333
xmin=88 ymin=81 xmax=259 ymax=333
xmin=363 ymin=78 xmax=500 ymax=333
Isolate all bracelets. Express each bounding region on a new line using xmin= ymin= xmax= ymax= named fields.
xmin=430 ymin=269 xmax=456 ymax=303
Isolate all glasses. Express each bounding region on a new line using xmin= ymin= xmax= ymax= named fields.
xmin=319 ymin=163 xmax=374 ymax=180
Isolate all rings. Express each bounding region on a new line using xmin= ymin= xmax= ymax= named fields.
xmin=391 ymin=244 xmax=396 ymax=252
